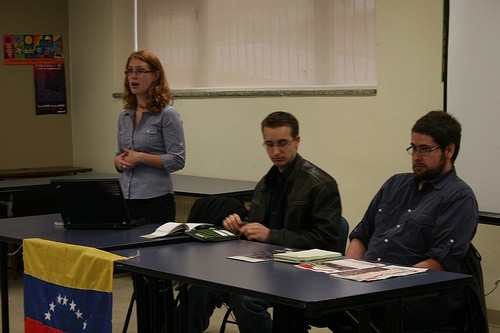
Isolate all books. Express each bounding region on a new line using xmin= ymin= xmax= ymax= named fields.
xmin=139 ymin=221 xmax=214 ymax=239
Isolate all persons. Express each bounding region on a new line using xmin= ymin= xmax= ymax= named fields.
xmin=162 ymin=111 xmax=342 ymax=333
xmin=272 ymin=111 xmax=479 ymax=333
xmin=113 ymin=51 xmax=185 ymax=333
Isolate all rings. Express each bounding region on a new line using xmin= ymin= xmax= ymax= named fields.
xmin=123 ymin=166 xmax=126 ymax=169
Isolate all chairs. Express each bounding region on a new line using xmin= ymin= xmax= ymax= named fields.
xmin=443 ymin=244 xmax=489 ymax=333
xmin=338 ymin=215 xmax=350 ymax=255
xmin=119 ymin=193 xmax=242 ymax=333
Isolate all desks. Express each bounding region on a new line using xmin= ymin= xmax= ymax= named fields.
xmin=0 ymin=172 xmax=120 ymax=281
xmin=0 ymin=211 xmax=192 ymax=333
xmin=0 ymin=165 xmax=94 ymax=176
xmin=88 ymin=172 xmax=260 ymax=225
xmin=108 ymin=238 xmax=474 ymax=333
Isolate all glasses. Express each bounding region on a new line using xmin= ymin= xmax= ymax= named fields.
xmin=125 ymin=68 xmax=154 ymax=75
xmin=405 ymin=144 xmax=439 ymax=156
xmin=263 ymin=138 xmax=294 ymax=148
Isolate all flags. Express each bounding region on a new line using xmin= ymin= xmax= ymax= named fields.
xmin=23 ymin=237 xmax=140 ymax=333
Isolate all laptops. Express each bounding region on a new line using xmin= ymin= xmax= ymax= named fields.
xmin=50 ymin=178 xmax=151 ymax=230
